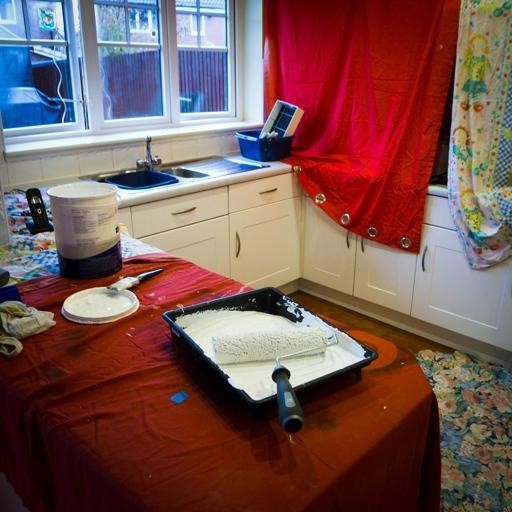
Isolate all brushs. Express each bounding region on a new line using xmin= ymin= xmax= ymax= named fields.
xmin=107 ymin=268 xmax=164 ymax=292
xmin=211 ymin=325 xmax=338 ymax=434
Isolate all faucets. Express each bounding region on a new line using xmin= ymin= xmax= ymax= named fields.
xmin=137 ymin=135 xmax=162 ymax=172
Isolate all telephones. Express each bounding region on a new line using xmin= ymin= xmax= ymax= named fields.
xmin=25 ymin=188 xmax=54 ymax=235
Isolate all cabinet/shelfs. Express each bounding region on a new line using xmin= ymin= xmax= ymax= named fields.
xmin=411 ymin=194 xmax=512 ymax=368
xmin=302 ymin=191 xmax=418 ymax=336
xmin=119 ymin=172 xmax=301 ymax=295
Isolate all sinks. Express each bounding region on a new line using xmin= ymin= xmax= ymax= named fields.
xmin=98 ymin=171 xmax=179 ymax=190
xmin=159 ymin=156 xmax=270 ymax=178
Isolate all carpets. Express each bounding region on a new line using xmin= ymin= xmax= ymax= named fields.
xmin=416 ymin=350 xmax=512 ymax=511
